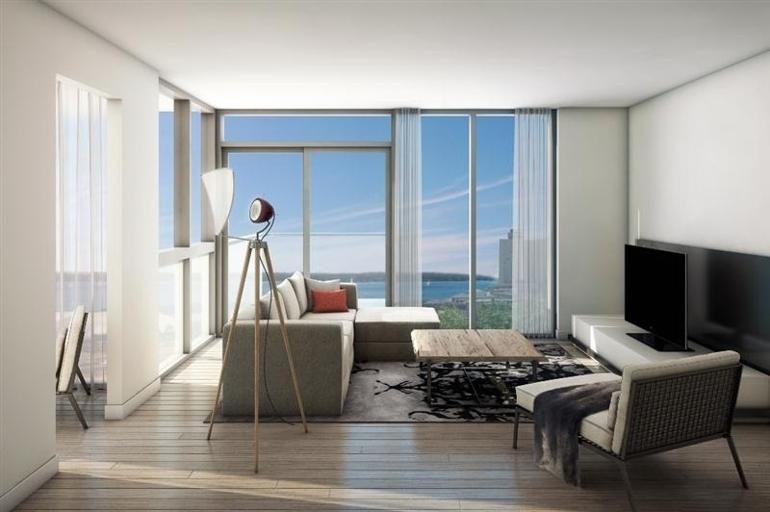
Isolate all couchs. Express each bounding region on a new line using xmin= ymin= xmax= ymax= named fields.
xmin=221 ymin=271 xmax=440 ymax=417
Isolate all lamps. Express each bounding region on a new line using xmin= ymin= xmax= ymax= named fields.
xmin=249 ymin=197 xmax=276 ymax=237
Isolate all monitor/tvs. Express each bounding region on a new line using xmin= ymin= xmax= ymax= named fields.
xmin=624 ymin=244 xmax=696 ymax=352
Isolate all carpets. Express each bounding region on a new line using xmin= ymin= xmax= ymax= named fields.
xmin=203 ymin=342 xmax=595 ymax=424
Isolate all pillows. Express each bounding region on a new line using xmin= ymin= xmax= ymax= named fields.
xmin=304 ymin=278 xmax=349 ymax=313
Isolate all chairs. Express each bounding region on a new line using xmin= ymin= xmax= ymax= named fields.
xmin=513 ymin=350 xmax=749 ymax=512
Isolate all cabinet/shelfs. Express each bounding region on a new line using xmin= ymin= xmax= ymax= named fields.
xmin=568 ymin=314 xmax=769 ymax=426
